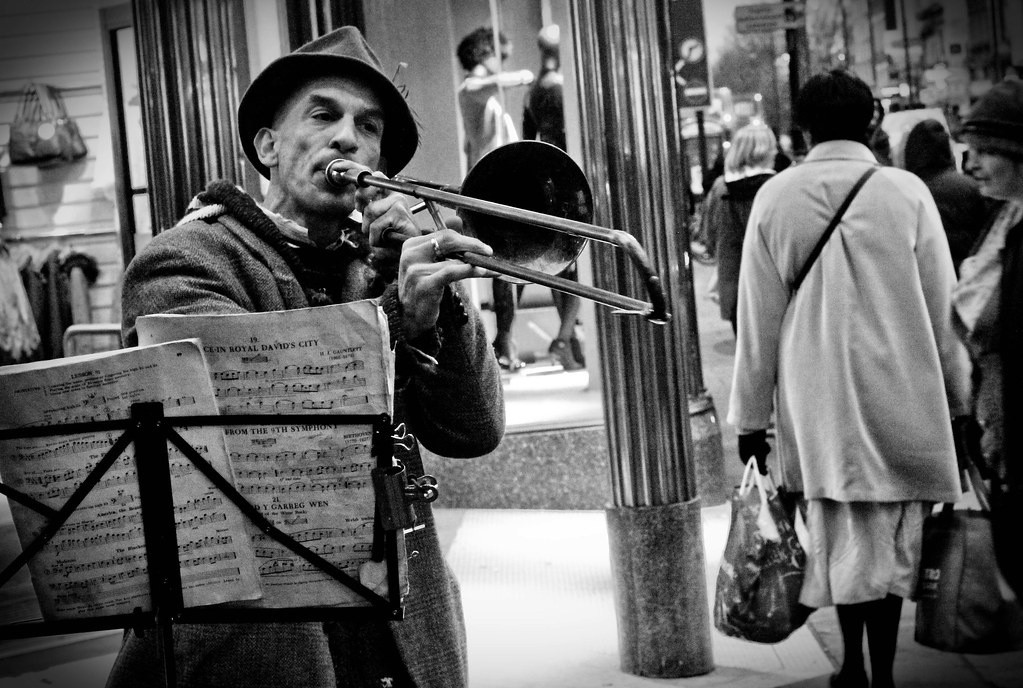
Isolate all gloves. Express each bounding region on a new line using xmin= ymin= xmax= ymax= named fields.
xmin=951 ymin=416 xmax=990 ymax=482
xmin=737 ymin=427 xmax=772 ymax=476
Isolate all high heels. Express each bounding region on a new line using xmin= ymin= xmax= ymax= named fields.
xmin=548 ymin=341 xmax=582 ymax=372
xmin=570 ymin=338 xmax=587 ymax=368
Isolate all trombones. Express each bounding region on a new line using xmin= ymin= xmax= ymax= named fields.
xmin=327 ymin=140 xmax=675 ymax=326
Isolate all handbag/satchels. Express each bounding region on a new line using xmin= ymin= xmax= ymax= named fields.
xmin=8 ymin=85 xmax=87 ymax=169
xmin=714 ymin=455 xmax=816 ymax=643
xmin=915 ymin=453 xmax=1023 ymax=655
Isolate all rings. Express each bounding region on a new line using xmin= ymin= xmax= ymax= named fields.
xmin=431 ymin=238 xmax=441 ymax=255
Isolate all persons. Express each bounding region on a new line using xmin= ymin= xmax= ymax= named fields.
xmin=727 ymin=68 xmax=973 ymax=688
xmin=456 ymin=28 xmax=534 ymax=369
xmin=902 ymin=119 xmax=1009 ymax=480
xmin=522 ymin=24 xmax=584 ymax=370
xmin=699 ymin=125 xmax=779 ymax=337
xmin=107 ymin=27 xmax=507 ymax=688
xmin=952 ymin=77 xmax=1022 ymax=604
xmin=872 ymin=125 xmax=892 ymax=166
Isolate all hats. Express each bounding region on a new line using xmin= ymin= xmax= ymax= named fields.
xmin=947 ymin=76 xmax=1023 ymax=148
xmin=239 ymin=26 xmax=420 ymax=180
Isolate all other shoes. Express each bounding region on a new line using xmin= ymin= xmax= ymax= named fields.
xmin=490 ymin=332 xmax=525 ymax=368
xmin=828 ymin=674 xmax=869 ymax=688
